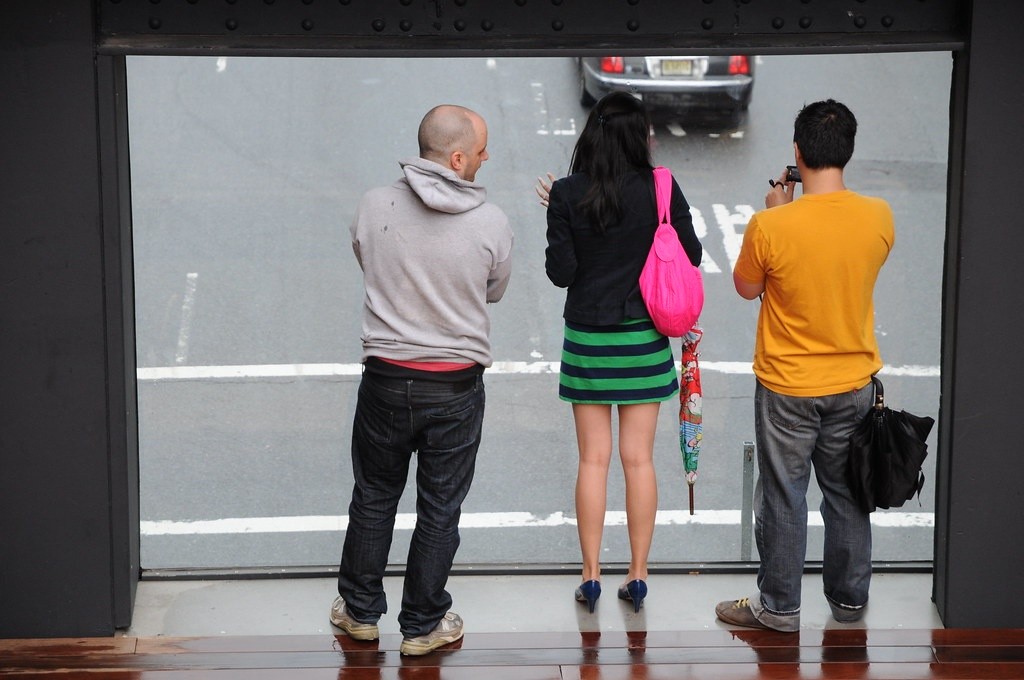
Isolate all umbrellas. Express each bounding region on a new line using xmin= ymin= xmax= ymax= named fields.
xmin=844 ymin=372 xmax=935 ymax=514
xmin=679 ymin=320 xmax=703 ymax=519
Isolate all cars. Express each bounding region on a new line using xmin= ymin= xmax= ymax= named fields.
xmin=573 ymin=56 xmax=754 ymax=132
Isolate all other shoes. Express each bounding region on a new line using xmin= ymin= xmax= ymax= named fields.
xmin=715 ymin=589 xmax=798 ymax=633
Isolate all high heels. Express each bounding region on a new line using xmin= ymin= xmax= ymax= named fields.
xmin=618 ymin=577 xmax=650 ymax=616
xmin=572 ymin=579 xmax=603 ymax=614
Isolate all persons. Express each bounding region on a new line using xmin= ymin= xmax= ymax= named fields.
xmin=535 ymin=91 xmax=703 ymax=612
xmin=711 ymin=97 xmax=896 ymax=633
xmin=331 ymin=103 xmax=513 ymax=655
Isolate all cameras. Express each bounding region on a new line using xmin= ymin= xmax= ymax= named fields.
xmin=786 ymin=165 xmax=801 ymax=182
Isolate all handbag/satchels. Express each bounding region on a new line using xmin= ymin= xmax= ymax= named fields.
xmin=640 ymin=160 xmax=702 ymax=336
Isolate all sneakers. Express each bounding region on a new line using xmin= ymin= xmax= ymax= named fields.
xmin=331 ymin=592 xmax=380 ymax=641
xmin=398 ymin=611 xmax=465 ymax=656
